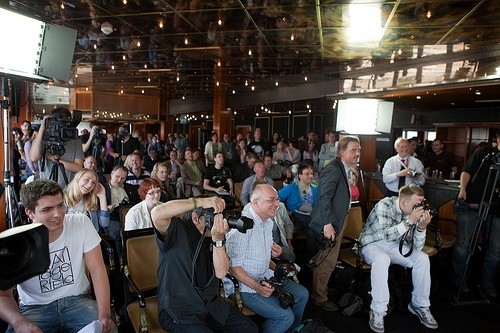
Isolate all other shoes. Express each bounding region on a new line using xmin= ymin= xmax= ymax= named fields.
xmin=312 ymin=300 xmax=339 ymax=311
xmin=482 ymin=280 xmax=497 ymax=297
xmin=456 ymin=279 xmax=469 ymax=292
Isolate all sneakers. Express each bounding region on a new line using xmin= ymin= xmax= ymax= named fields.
xmin=369 ymin=310 xmax=387 ymax=333
xmin=408 ymin=302 xmax=438 ymax=329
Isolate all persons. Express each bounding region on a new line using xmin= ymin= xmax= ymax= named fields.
xmin=251 ymin=180 xmax=300 ymax=284
xmin=454 ymin=133 xmax=500 ymax=297
xmin=352 ymin=184 xmax=439 ymax=333
xmin=29 ymin=107 xmax=86 ymax=192
xmin=276 ymin=161 xmax=318 ymax=236
xmin=12 ymin=120 xmax=338 ymax=202
xmin=151 ymin=197 xmax=260 ymax=333
xmin=382 ymin=137 xmax=426 ymax=198
xmin=308 ymin=136 xmax=362 ymax=312
xmin=125 ymin=178 xmax=165 ymax=232
xmin=0 ymin=178 xmax=119 ymax=333
xmin=407 ymin=138 xmax=458 ymax=176
xmin=98 ymin=165 xmax=138 ymax=268
xmin=224 ymin=184 xmax=309 ymax=333
xmin=62 ymin=168 xmax=121 ymax=326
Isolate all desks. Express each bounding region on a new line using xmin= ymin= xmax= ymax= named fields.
xmin=367 ymin=172 xmax=482 ymax=247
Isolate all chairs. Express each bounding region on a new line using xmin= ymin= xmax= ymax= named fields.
xmin=98 ymin=174 xmax=459 ymax=333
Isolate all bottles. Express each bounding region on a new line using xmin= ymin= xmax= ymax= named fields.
xmin=375 ymin=160 xmax=385 ymax=173
xmin=424 ymin=167 xmax=430 ymax=179
xmin=431 ymin=169 xmax=443 ymax=182
xmin=450 ymin=171 xmax=457 ymax=180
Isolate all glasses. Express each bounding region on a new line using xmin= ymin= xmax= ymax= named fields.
xmin=257 ymin=197 xmax=280 ymax=202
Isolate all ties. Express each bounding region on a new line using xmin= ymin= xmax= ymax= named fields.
xmin=398 ymin=160 xmax=405 ymax=189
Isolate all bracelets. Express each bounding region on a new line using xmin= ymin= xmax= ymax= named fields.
xmin=404 ymin=219 xmax=411 ymax=227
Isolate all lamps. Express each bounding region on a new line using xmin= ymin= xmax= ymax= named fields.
xmin=101 ymin=22 xmax=113 ymax=34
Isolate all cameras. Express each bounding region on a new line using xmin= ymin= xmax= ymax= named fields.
xmin=267 ymin=267 xmax=295 ymax=310
xmin=205 ymin=209 xmax=254 ymax=234
xmin=416 ymin=199 xmax=438 ymax=216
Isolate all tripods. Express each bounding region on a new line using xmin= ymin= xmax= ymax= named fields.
xmin=452 ymin=151 xmax=500 ymax=305
xmin=93 ymin=142 xmax=106 ymax=174
xmin=48 ymin=155 xmax=68 ymax=186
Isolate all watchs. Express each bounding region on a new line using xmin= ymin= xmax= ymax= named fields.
xmin=211 ymin=238 xmax=226 ymax=247
xmin=189 ymin=197 xmax=196 ymax=209
